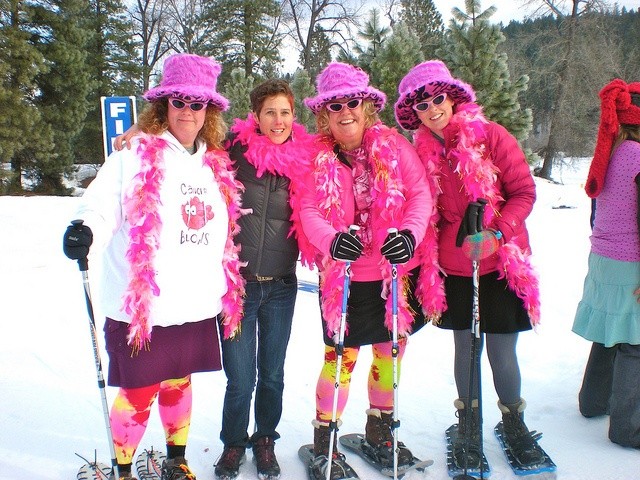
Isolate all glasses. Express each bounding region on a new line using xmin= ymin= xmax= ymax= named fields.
xmin=412 ymin=92 xmax=447 ymax=113
xmin=326 ymin=98 xmax=363 ymax=112
xmin=167 ymin=97 xmax=208 ymax=112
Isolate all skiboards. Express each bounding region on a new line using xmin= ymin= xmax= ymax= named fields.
xmin=77 ymin=449 xmax=167 ymax=480
xmin=445 ymin=422 xmax=557 ymax=479
xmin=299 ymin=434 xmax=432 ymax=479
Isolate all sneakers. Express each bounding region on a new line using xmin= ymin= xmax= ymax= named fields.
xmin=252 ymin=436 xmax=280 ymax=480
xmin=213 ymin=446 xmax=247 ymax=480
xmin=161 ymin=457 xmax=196 ymax=480
xmin=109 ymin=471 xmax=137 ymax=480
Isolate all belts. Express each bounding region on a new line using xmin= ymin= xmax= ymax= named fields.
xmin=242 ymin=275 xmax=274 ymax=282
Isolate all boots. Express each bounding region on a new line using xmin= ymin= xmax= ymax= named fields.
xmin=366 ymin=409 xmax=413 ymax=468
xmin=311 ymin=419 xmax=347 ymax=478
xmin=497 ymin=397 xmax=545 ymax=466
xmin=454 ymin=399 xmax=481 ymax=470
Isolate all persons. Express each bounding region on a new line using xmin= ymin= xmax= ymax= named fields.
xmin=395 ymin=59 xmax=544 ymax=468
xmin=210 ymin=79 xmax=311 ymax=480
xmin=569 ymin=79 xmax=639 ymax=448
xmin=62 ymin=52 xmax=256 ymax=480
xmin=299 ymin=62 xmax=431 ymax=480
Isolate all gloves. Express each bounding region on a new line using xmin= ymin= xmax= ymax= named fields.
xmin=381 ymin=229 xmax=416 ymax=264
xmin=63 ymin=225 xmax=93 ymax=259
xmin=462 ymin=227 xmax=505 ymax=260
xmin=330 ymin=231 xmax=364 ymax=262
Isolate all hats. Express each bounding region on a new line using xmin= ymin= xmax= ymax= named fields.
xmin=394 ymin=60 xmax=476 ymax=131
xmin=304 ymin=62 xmax=387 ymax=116
xmin=143 ymin=54 xmax=228 ymax=112
